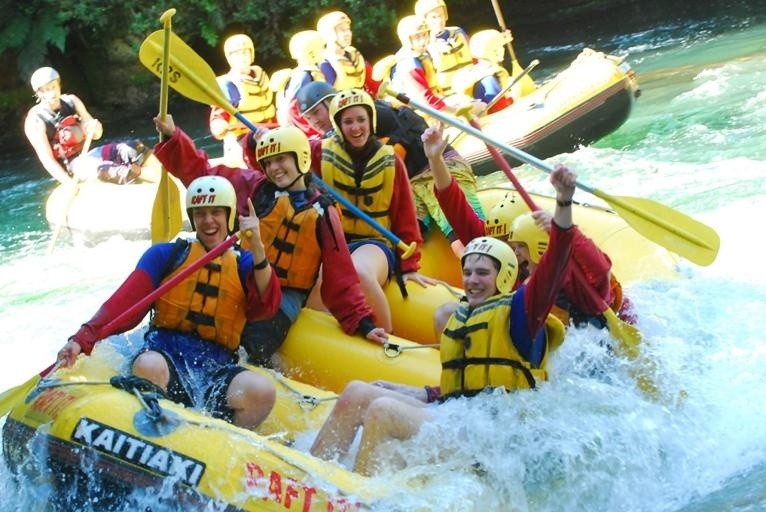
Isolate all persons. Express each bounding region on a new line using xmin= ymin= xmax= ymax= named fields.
xmin=253 ymin=89 xmax=436 ymax=335
xmin=416 ymin=1 xmax=472 ymax=97
xmin=275 ymin=30 xmax=327 ymax=144
xmin=313 ymin=11 xmax=384 ymax=98
xmin=421 ymin=120 xmax=640 ymax=360
xmin=57 ymin=176 xmax=281 ymax=430
xmin=153 ymin=113 xmax=389 ymax=368
xmin=211 ymin=34 xmax=274 ymax=169
xmin=373 ymin=15 xmax=484 ymax=120
xmin=310 ymin=163 xmax=578 ymax=478
xmin=24 ymin=66 xmax=161 ymax=185
xmin=454 ymin=30 xmax=512 ymax=115
xmin=297 ymin=81 xmax=487 ymax=258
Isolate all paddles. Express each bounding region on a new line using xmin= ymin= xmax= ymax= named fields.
xmin=139 ymin=30 xmax=416 ymax=260
xmin=491 ymin=1 xmax=537 ymax=99
xmin=0 ymin=230 xmax=251 ymax=417
xmin=378 ymin=80 xmax=719 ymax=266
xmin=457 ymin=106 xmax=688 ymax=405
xmin=152 ymin=8 xmax=181 ymax=242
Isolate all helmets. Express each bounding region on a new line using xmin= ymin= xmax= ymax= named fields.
xmin=484 ymin=199 xmax=531 ymax=237
xmin=508 ymin=212 xmax=550 ymax=264
xmin=221 ymin=0 xmax=508 ymax=68
xmin=256 ymin=126 xmax=312 ymax=174
xmin=329 ymin=89 xmax=377 ymax=142
xmin=185 ymin=175 xmax=237 ymax=231
xmin=31 ymin=67 xmax=60 ymax=92
xmin=297 ymin=81 xmax=337 ymax=117
xmin=460 ymin=236 xmax=518 ymax=293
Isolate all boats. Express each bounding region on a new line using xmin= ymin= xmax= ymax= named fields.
xmin=41 ymin=48 xmax=644 ymax=236
xmin=0 ymin=185 xmax=680 ymax=512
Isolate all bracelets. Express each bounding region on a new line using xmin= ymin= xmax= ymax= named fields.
xmin=555 ymin=198 xmax=572 ymax=206
xmin=252 ymin=257 xmax=268 ymax=270
